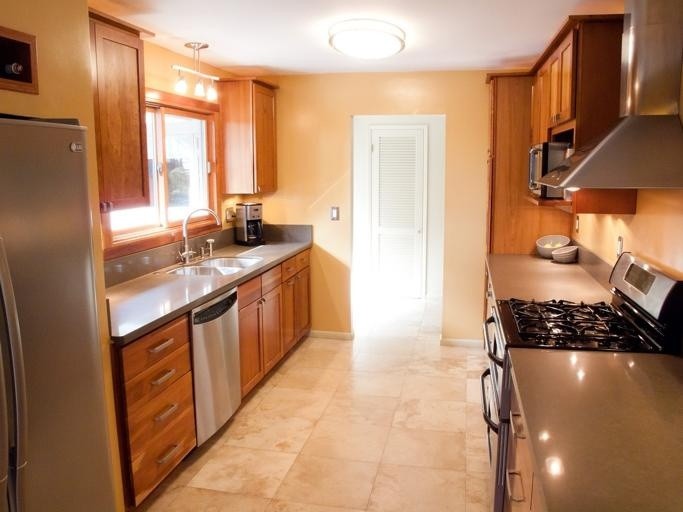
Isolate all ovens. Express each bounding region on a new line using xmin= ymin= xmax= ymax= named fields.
xmin=480 ymin=316 xmax=512 ymax=508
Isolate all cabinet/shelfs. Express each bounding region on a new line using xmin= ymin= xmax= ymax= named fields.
xmin=217 ymin=77 xmax=278 ymax=195
xmin=119 ymin=312 xmax=198 ymax=509
xmin=90 ymin=8 xmax=155 ymax=211
xmin=501 ymin=348 xmax=683 ymax=507
xmin=485 ymin=73 xmax=575 ymax=252
xmin=525 ymin=14 xmax=635 ymax=214
xmin=238 ymin=246 xmax=313 ymax=402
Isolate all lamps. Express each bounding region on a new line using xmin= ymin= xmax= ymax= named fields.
xmin=174 ymin=42 xmax=221 ymax=103
xmin=319 ymin=22 xmax=408 ymax=63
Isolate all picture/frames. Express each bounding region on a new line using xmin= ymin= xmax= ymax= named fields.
xmin=0 ymin=26 xmax=38 ymax=94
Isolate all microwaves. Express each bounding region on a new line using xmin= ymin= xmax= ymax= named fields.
xmin=529 ymin=143 xmax=571 ymax=198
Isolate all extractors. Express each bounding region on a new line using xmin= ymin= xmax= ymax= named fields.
xmin=534 ymin=0 xmax=682 ymax=189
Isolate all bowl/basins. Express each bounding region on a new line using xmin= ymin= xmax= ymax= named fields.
xmin=552 ymin=245 xmax=578 ymax=263
xmin=535 ymin=235 xmax=570 ymax=257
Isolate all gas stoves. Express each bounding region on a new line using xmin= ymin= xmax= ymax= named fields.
xmin=492 ymin=251 xmax=683 ymax=352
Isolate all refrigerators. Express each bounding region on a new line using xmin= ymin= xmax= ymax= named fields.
xmin=0 ymin=116 xmax=126 ymax=512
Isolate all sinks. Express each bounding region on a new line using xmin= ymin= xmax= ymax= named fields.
xmin=196 ymin=255 xmax=266 ymax=268
xmin=166 ymin=265 xmax=244 ymax=278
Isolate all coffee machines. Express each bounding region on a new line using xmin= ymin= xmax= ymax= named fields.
xmin=236 ymin=202 xmax=263 ymax=245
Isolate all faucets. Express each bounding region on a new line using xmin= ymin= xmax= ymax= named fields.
xmin=183 ymin=208 xmax=221 ymax=265
